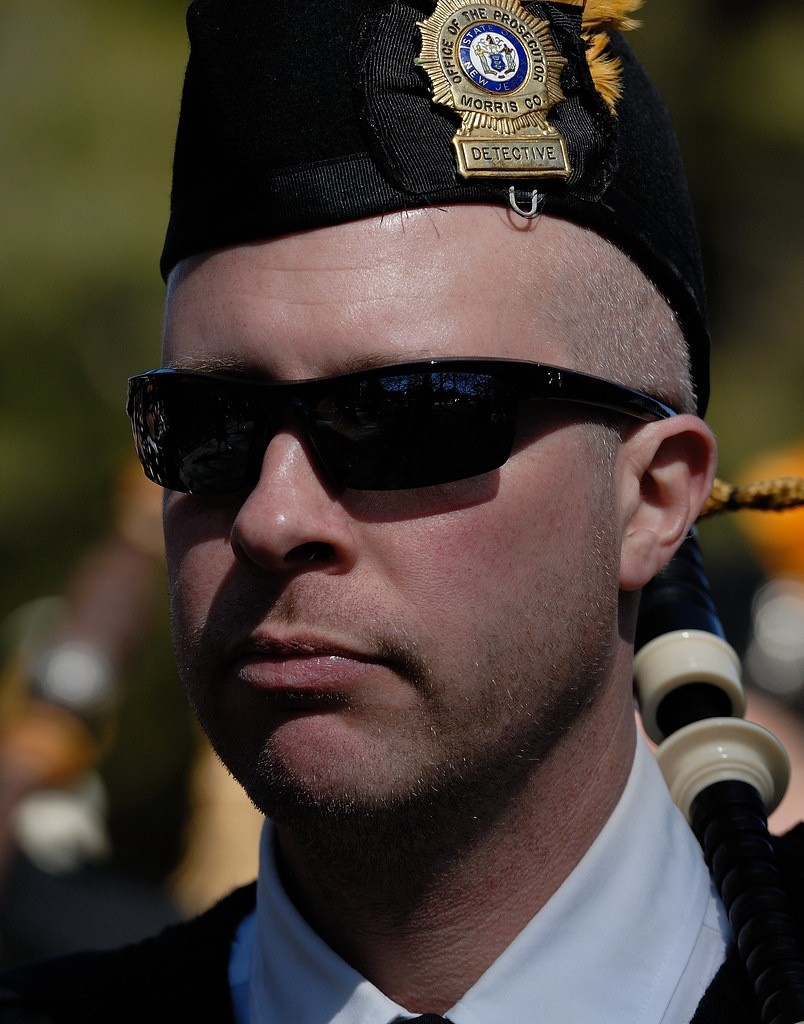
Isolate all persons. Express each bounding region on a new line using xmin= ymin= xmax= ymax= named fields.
xmin=0 ymin=414 xmax=803 ymax=1023
xmin=1 ymin=0 xmax=804 ymax=1024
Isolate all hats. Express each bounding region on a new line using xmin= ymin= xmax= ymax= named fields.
xmin=160 ymin=0 xmax=711 ymax=420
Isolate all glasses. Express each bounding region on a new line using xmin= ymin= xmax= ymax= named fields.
xmin=126 ymin=357 xmax=678 ymax=496
